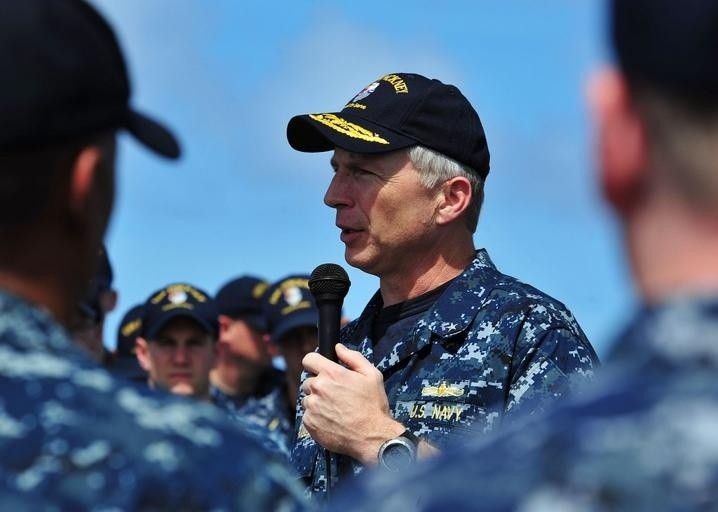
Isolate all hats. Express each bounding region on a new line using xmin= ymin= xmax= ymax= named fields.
xmin=287 ymin=73 xmax=489 ymax=179
xmin=1 ymin=0 xmax=180 ymax=161
xmin=117 ymin=275 xmax=319 ymax=381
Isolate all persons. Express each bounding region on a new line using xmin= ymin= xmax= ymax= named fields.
xmin=106 ymin=268 xmax=353 ymax=461
xmin=340 ymin=2 xmax=717 ymax=508
xmin=0 ymin=2 xmax=321 ymax=510
xmin=286 ymin=69 xmax=602 ymax=499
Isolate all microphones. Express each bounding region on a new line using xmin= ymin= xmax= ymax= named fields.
xmin=309 ymin=264 xmax=350 ymax=364
